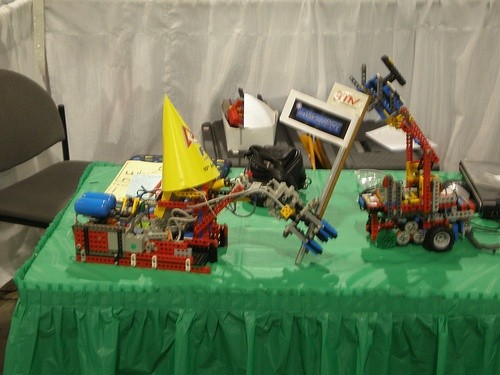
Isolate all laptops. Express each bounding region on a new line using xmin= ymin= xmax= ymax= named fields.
xmin=458 ymin=160 xmax=500 ymax=217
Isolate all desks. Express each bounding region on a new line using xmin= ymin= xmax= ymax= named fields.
xmin=323 ymin=121 xmax=441 ymax=171
xmin=209 ymin=119 xmax=299 ymax=168
xmin=2 ymin=163 xmax=500 ymax=375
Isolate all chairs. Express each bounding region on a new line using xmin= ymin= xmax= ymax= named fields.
xmin=0 ymin=67 xmax=92 ymax=229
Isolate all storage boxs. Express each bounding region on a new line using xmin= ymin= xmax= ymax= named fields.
xmin=222 ymin=93 xmax=279 ymax=151
